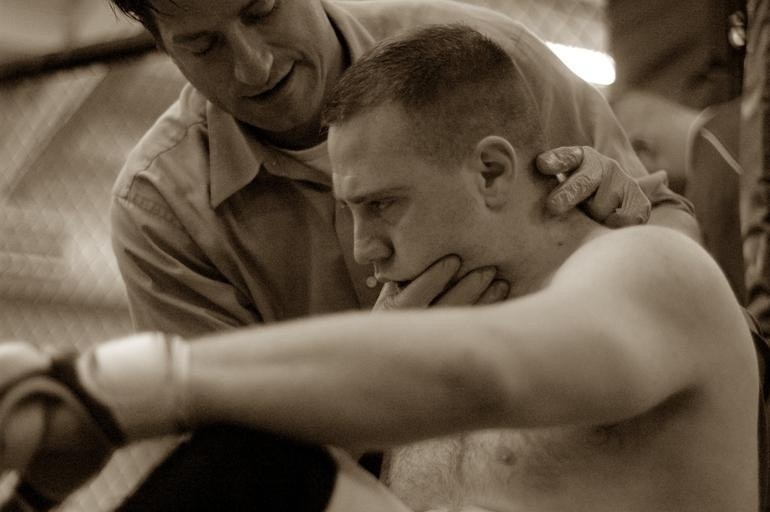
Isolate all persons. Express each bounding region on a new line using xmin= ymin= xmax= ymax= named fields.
xmin=109 ymin=0 xmax=699 ymax=512
xmin=0 ymin=25 xmax=759 ymax=512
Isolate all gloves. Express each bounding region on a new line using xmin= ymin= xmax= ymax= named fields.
xmin=0 ymin=332 xmax=185 ymax=512
